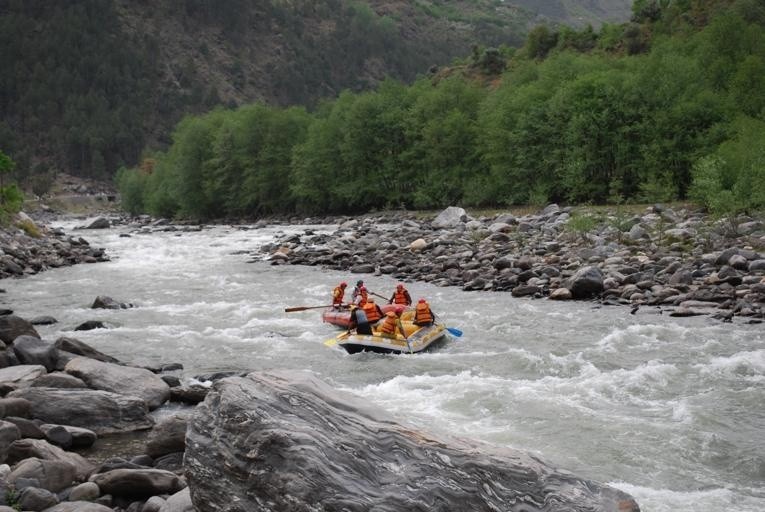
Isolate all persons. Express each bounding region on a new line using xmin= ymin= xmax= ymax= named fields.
xmin=332 ymin=282 xmax=351 ymax=309
xmin=389 ymin=284 xmax=412 ymax=307
xmin=348 ymin=305 xmax=374 ymax=336
xmin=413 ymin=298 xmax=435 ymax=327
xmin=380 ymin=307 xmax=407 ymax=339
xmin=362 ymin=298 xmax=384 ymax=327
xmin=353 ymin=280 xmax=368 ymax=307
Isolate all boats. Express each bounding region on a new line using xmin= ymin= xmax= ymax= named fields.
xmin=321 ymin=302 xmax=412 ymax=329
xmin=325 ymin=310 xmax=447 ymax=356
